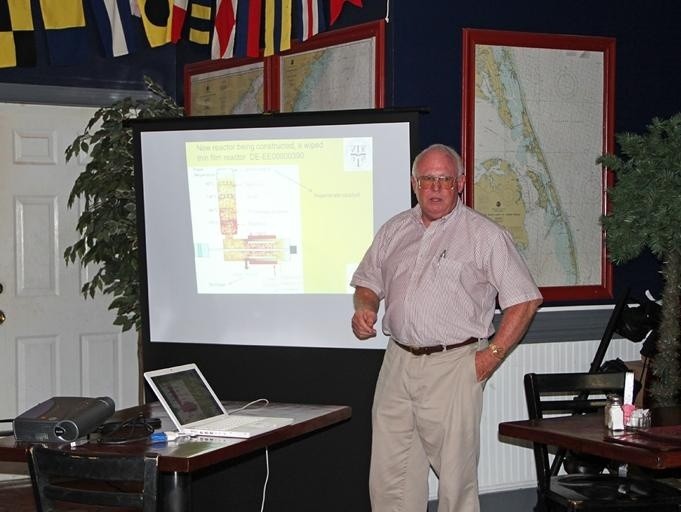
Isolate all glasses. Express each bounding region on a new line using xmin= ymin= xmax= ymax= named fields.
xmin=417 ymin=175 xmax=460 ymax=189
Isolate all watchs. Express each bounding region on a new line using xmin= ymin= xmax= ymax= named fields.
xmin=488 ymin=343 xmax=507 ymax=361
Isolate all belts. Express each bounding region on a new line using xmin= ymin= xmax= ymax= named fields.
xmin=395 ymin=339 xmax=480 ymax=355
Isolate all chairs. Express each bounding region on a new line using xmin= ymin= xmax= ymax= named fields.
xmin=524 ymin=372 xmax=681 ymax=512
xmin=25 ymin=445 xmax=158 ymax=512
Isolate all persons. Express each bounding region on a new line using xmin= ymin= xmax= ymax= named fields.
xmin=349 ymin=145 xmax=543 ymax=511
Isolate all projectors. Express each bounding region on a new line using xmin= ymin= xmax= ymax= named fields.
xmin=13 ymin=397 xmax=114 ymax=445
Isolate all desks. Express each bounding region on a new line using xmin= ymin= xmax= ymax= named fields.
xmin=0 ymin=398 xmax=352 ymax=512
xmin=498 ymin=412 xmax=681 ymax=512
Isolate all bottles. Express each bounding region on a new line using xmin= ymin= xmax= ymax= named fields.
xmin=604 ymin=393 xmax=624 ymax=432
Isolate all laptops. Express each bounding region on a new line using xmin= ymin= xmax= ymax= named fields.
xmin=144 ymin=363 xmax=293 ymax=438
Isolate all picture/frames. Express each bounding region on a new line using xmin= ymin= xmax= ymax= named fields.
xmin=184 ymin=47 xmax=272 ymax=118
xmin=271 ymin=19 xmax=386 ymax=113
xmin=462 ymin=27 xmax=616 ymax=302
